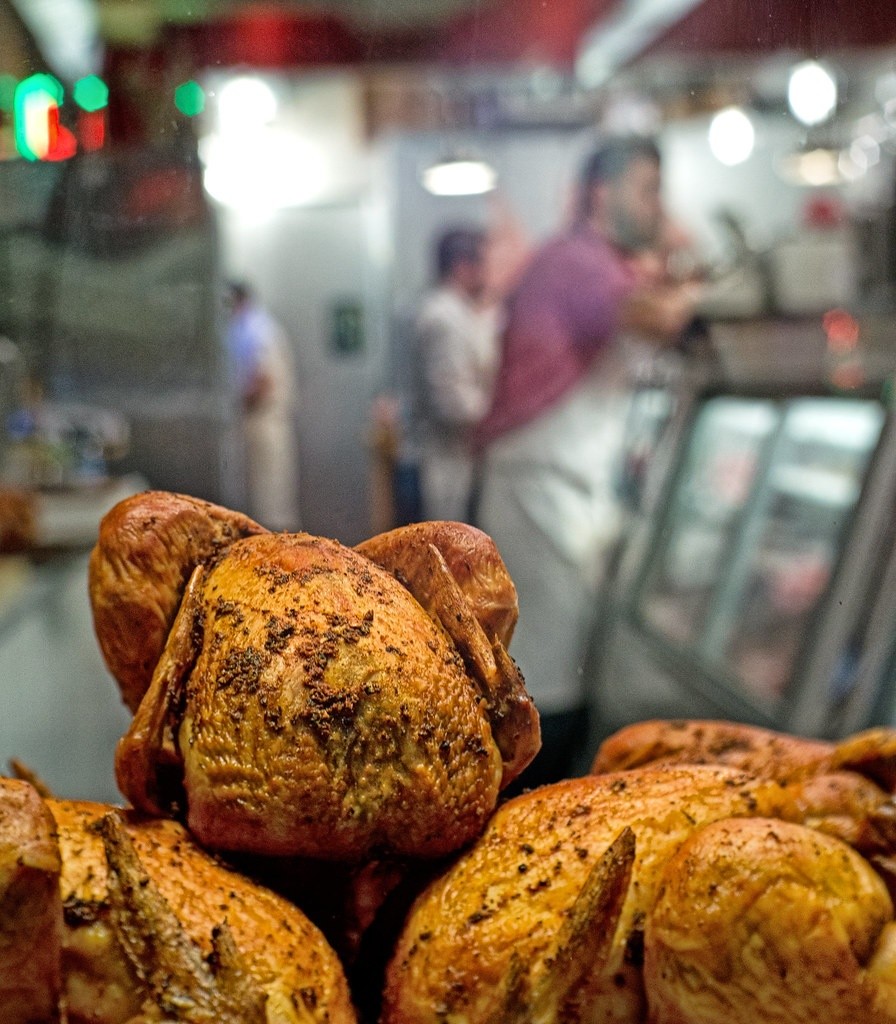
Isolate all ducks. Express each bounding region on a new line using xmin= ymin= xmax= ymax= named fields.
xmin=0 ymin=752 xmax=358 ymax=1023
xmin=376 ymin=713 xmax=895 ymax=1024
xmin=89 ymin=484 xmax=544 ymax=944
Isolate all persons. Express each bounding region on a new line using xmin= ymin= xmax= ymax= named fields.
xmin=387 ymin=224 xmax=513 ymax=528
xmin=473 ymin=133 xmax=766 ymax=777
xmin=225 ymin=277 xmax=308 ymax=535
